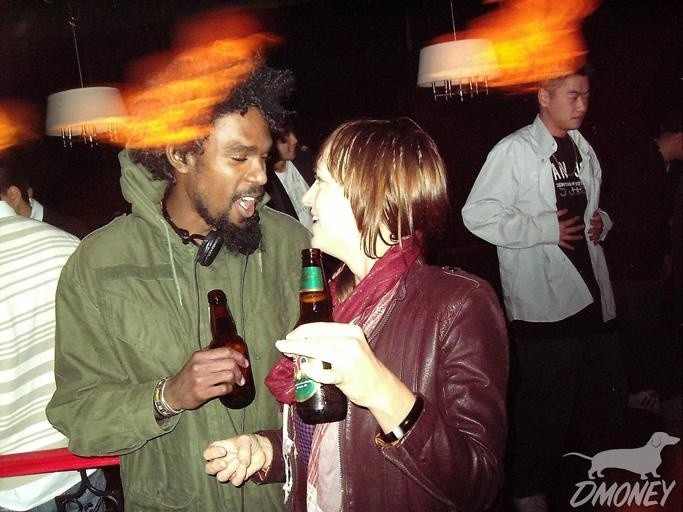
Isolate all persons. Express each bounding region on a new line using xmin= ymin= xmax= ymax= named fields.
xmin=0 ymin=162 xmax=109 ymax=240
xmin=248 ymin=102 xmax=338 ymax=281
xmin=43 ymin=58 xmax=321 ymax=511
xmin=202 ymin=110 xmax=511 ymax=512
xmin=0 ymin=194 xmax=111 ymax=511
xmin=580 ymin=121 xmax=681 ymax=424
xmin=458 ymin=67 xmax=615 ymax=512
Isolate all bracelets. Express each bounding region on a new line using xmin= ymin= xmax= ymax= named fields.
xmin=152 ymin=376 xmax=184 ymax=421
xmin=373 ymin=390 xmax=425 ymax=444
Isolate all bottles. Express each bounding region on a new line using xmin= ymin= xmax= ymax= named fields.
xmin=291 ymin=247 xmax=346 ymax=425
xmin=209 ymin=289 xmax=256 ymax=409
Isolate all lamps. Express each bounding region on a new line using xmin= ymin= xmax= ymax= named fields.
xmin=416 ymin=1 xmax=501 ymax=106
xmin=42 ymin=6 xmax=128 ymax=150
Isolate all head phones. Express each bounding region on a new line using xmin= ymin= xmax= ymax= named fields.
xmin=162 ymin=182 xmax=262 ymax=267
